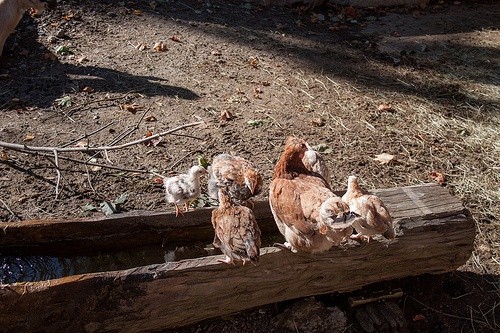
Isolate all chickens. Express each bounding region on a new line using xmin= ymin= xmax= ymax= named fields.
xmin=163 ymin=165 xmax=209 ymax=218
xmin=283 ymin=135 xmax=328 ymax=186
xmin=206 ymin=153 xmax=263 ymax=210
xmin=268 ymin=139 xmax=362 ymax=254
xmin=340 ymin=175 xmax=396 ymax=243
xmin=211 ymin=188 xmax=262 ymax=267
